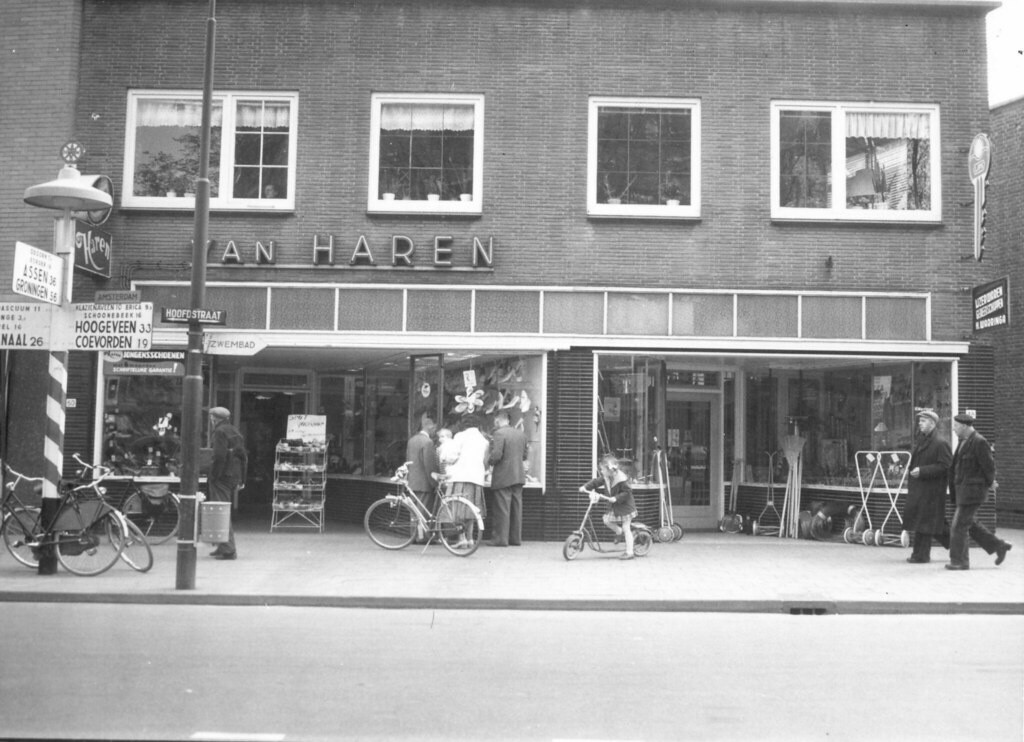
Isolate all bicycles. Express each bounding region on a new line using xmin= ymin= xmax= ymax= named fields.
xmin=564 ymin=488 xmax=655 ymax=560
xmin=0 ymin=453 xmax=181 ymax=576
xmin=364 ymin=461 xmax=485 ymax=557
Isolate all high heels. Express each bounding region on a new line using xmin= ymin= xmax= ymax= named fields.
xmin=451 ymin=540 xmax=467 ymax=548
xmin=468 ymin=540 xmax=474 ymax=548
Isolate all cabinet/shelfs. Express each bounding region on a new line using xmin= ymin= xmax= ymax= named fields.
xmin=269 ymin=438 xmax=331 ymax=534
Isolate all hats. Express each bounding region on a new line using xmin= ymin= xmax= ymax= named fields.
xmin=210 ymin=407 xmax=230 ymax=418
xmin=916 ymin=411 xmax=940 ymax=425
xmin=955 ymin=414 xmax=974 ymax=426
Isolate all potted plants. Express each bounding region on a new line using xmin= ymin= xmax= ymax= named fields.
xmin=378 ymin=166 xmax=409 ymax=200
xmin=155 ymin=169 xmax=190 ymax=197
xmin=422 ymin=173 xmax=449 ymax=201
xmin=870 ymin=162 xmax=897 ymax=210
xmin=449 ymin=156 xmax=472 ymax=201
xmin=598 ymin=171 xmax=639 ymax=204
xmin=657 ymin=172 xmax=685 ymax=205
xmin=183 ymin=166 xmax=219 ymax=197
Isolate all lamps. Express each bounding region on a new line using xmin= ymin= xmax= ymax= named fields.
xmin=825 ymin=256 xmax=834 ymax=269
xmin=874 ymin=422 xmax=889 ymax=447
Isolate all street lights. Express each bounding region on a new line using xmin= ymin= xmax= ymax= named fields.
xmin=24 ymin=166 xmax=113 ymax=576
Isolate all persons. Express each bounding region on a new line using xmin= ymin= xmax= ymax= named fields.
xmin=486 ymin=412 xmax=527 ymax=546
xmin=945 ymin=415 xmax=1012 ymax=570
xmin=207 ymin=407 xmax=248 ymax=559
xmin=578 ymin=454 xmax=638 ymax=560
xmin=405 ymin=412 xmax=442 ymax=547
xmin=435 ymin=415 xmax=490 ymax=550
xmin=265 ymin=184 xmax=279 ymax=197
xmin=903 ymin=411 xmax=951 ymax=565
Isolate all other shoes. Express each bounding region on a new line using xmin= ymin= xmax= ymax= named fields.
xmin=945 ymin=562 xmax=970 ymax=570
xmin=209 ymin=548 xmax=221 ymax=556
xmin=620 ymin=553 xmax=634 ymax=560
xmin=216 ymin=553 xmax=237 ymax=560
xmin=907 ymin=558 xmax=928 ymax=563
xmin=995 ymin=542 xmax=1011 ymax=565
xmin=614 ymin=531 xmax=624 ymax=544
